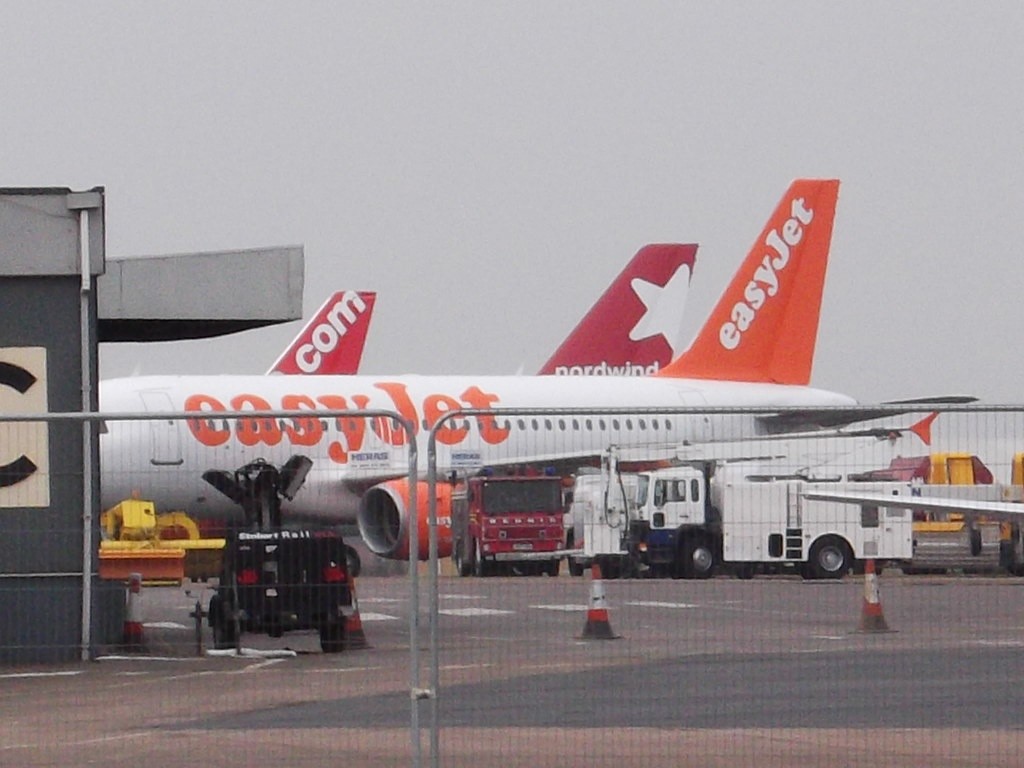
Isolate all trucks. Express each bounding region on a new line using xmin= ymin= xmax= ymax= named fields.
xmin=450 ymin=475 xmax=564 ymax=577
xmin=628 ymin=464 xmax=913 ymax=580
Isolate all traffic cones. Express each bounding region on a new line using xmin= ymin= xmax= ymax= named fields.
xmin=120 ymin=572 xmax=151 ymax=654
xmin=848 ymin=558 xmax=899 ymax=634
xmin=574 ymin=564 xmax=622 ymax=640
xmin=95 ymin=178 xmax=1023 ymax=561
xmin=333 ymin=568 xmax=374 ymax=652
